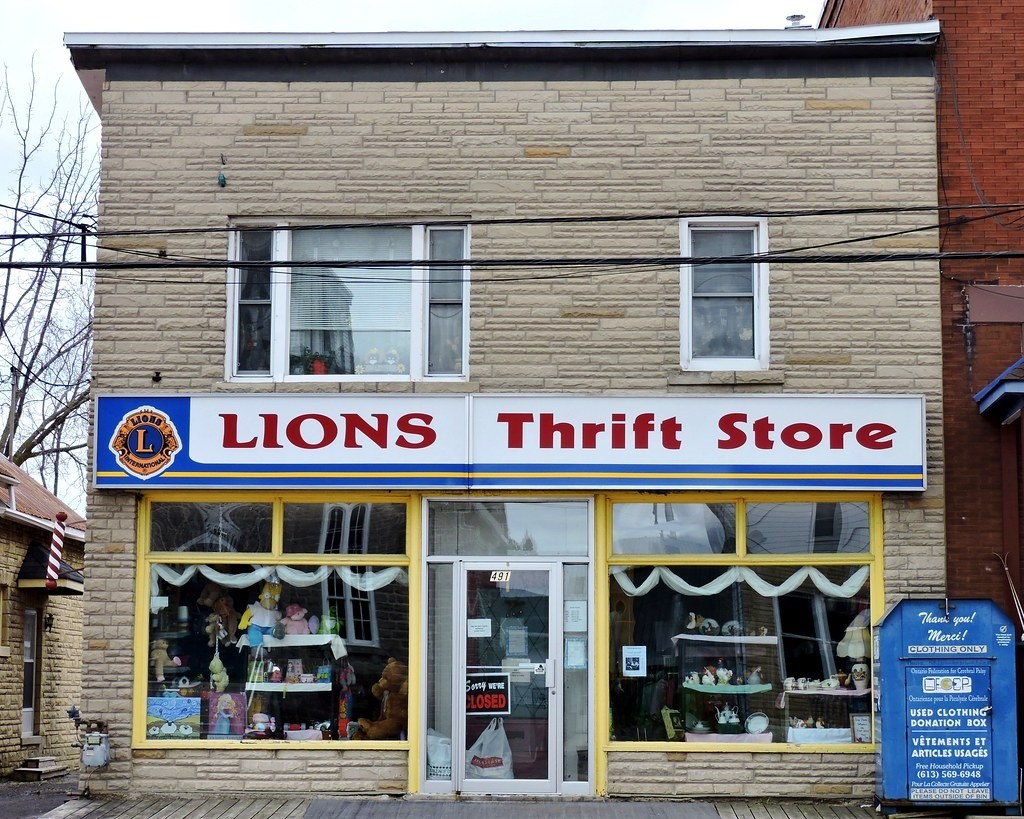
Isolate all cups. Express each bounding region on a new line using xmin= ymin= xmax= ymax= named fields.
xmin=719 ymin=717 xmax=725 ymax=722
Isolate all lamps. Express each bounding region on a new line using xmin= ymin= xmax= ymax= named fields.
xmin=44 ymin=613 xmax=54 ymax=633
xmin=837 ymin=627 xmax=871 ymax=689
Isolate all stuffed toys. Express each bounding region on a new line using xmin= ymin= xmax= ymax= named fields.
xmin=148 ymin=569 xmax=410 ymax=740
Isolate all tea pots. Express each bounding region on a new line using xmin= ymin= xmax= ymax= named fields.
xmin=830 ymin=669 xmax=851 ymax=690
xmin=693 ymin=720 xmax=703 ymax=728
xmin=715 ymin=702 xmax=738 ymax=723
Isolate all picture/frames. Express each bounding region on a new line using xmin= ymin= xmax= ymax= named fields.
xmin=849 ymin=712 xmax=872 ymax=743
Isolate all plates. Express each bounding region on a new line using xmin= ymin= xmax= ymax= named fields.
xmin=744 ymin=713 xmax=769 ymax=734
xmin=693 ymin=727 xmax=710 ymax=730
xmin=718 ymin=721 xmax=727 ymax=724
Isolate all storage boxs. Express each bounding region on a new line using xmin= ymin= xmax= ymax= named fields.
xmin=208 ymin=691 xmax=245 ymax=735
xmin=146 ymin=697 xmax=201 ymax=740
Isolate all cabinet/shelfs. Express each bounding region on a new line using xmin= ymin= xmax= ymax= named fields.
xmin=672 ymin=633 xmax=778 ymax=743
xmin=235 ymin=634 xmax=348 ymax=740
